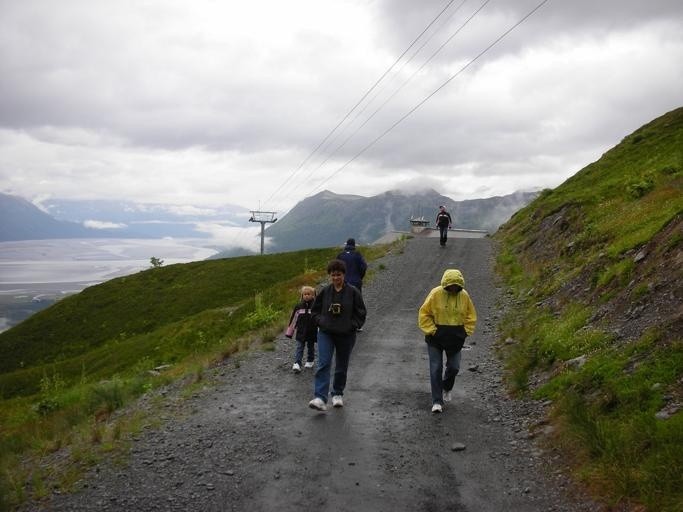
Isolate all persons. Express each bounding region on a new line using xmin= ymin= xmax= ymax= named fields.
xmin=310 ymin=260 xmax=366 ymax=411
xmin=286 ymin=285 xmax=317 ymax=372
xmin=336 ymin=238 xmax=367 ymax=331
xmin=436 ymin=205 xmax=452 ymax=248
xmin=418 ymin=269 xmax=477 ymax=413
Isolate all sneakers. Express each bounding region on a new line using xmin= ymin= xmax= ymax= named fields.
xmin=291 ymin=362 xmax=303 ymax=373
xmin=303 ymin=360 xmax=315 ymax=368
xmin=309 ymin=397 xmax=329 ymax=412
xmin=355 ymin=326 xmax=364 ymax=331
xmin=331 ymin=393 xmax=344 ymax=407
xmin=432 ymin=403 xmax=443 ymax=413
xmin=442 ymin=387 xmax=453 ymax=402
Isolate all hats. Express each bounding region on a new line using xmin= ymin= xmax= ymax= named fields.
xmin=438 ymin=205 xmax=447 ymax=211
